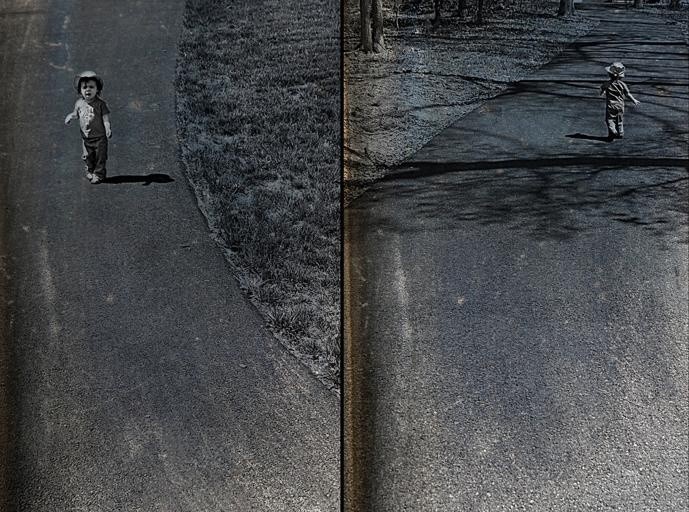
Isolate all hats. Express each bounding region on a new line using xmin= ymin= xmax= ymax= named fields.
xmin=605 ymin=62 xmax=625 ymax=78
xmin=73 ymin=71 xmax=103 ymax=93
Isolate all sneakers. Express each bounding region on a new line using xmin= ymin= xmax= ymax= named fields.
xmin=608 ymin=132 xmax=624 ymax=142
xmin=85 ymin=173 xmax=105 ymax=184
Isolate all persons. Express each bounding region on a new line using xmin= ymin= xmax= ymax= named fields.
xmin=65 ymin=71 xmax=112 ymax=184
xmin=600 ymin=62 xmax=641 ymax=141
